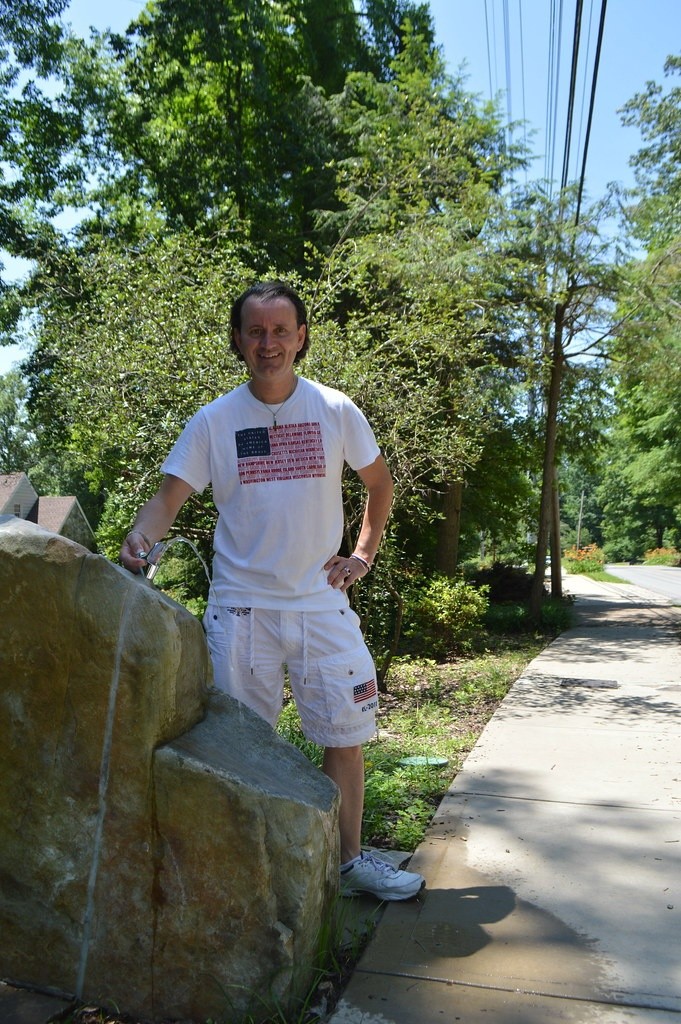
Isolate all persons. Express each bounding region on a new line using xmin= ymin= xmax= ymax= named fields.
xmin=120 ymin=282 xmax=426 ymax=902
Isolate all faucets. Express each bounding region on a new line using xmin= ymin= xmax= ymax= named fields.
xmin=139 ymin=542 xmax=161 ymax=582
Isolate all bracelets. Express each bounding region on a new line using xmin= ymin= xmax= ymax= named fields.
xmin=126 ymin=530 xmax=151 ymax=550
xmin=349 ymin=553 xmax=371 ymax=573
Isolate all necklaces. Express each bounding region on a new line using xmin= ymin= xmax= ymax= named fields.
xmin=249 ymin=374 xmax=300 ymax=433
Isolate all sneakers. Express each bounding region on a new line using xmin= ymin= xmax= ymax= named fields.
xmin=340 ymin=851 xmax=426 ymax=901
